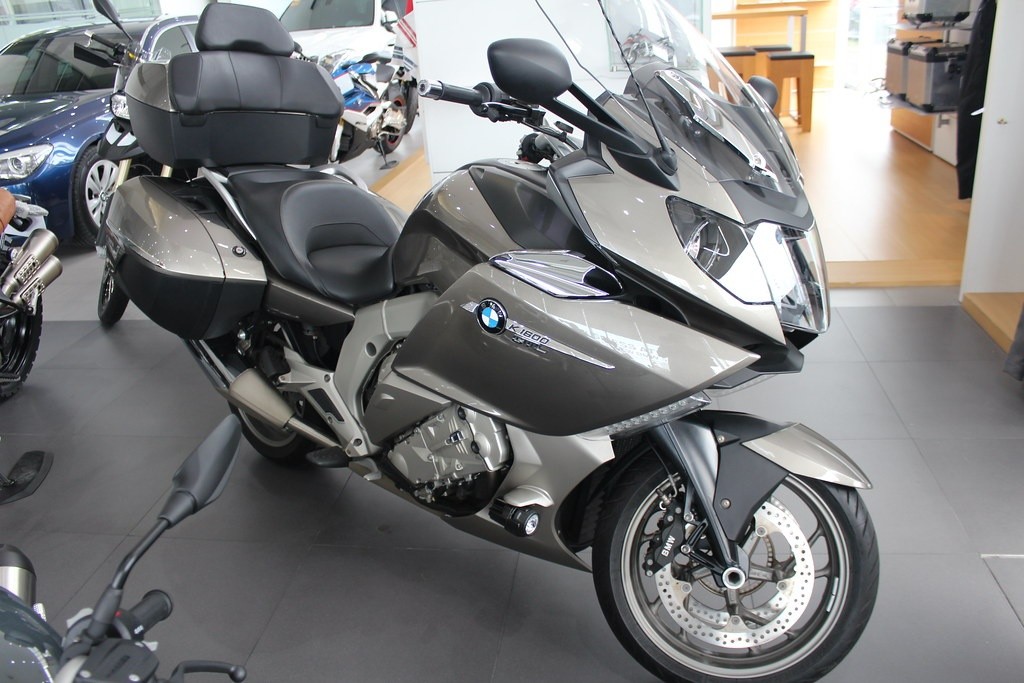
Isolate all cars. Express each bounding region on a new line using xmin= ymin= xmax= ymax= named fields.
xmin=0 ymin=12 xmax=200 ymax=249
xmin=273 ymin=0 xmax=422 ymax=135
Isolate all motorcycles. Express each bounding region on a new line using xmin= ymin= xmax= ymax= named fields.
xmin=620 ymin=28 xmax=674 ymax=66
xmin=292 ymin=43 xmax=407 ymax=169
xmin=103 ymin=0 xmax=880 ymax=683
xmin=0 ymin=187 xmax=63 ymax=401
xmin=0 ymin=415 xmax=248 ymax=683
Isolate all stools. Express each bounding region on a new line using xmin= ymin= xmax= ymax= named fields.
xmin=753 ymin=45 xmax=792 ymax=117
xmin=766 ymin=51 xmax=814 ymax=134
xmin=707 ymin=47 xmax=756 ymax=111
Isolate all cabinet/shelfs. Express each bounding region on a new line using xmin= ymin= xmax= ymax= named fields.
xmin=959 ymin=0 xmax=1024 ymax=373
xmin=887 ymin=94 xmax=958 ymax=166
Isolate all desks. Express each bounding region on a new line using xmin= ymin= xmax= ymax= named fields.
xmin=711 ymin=6 xmax=808 ymax=53
